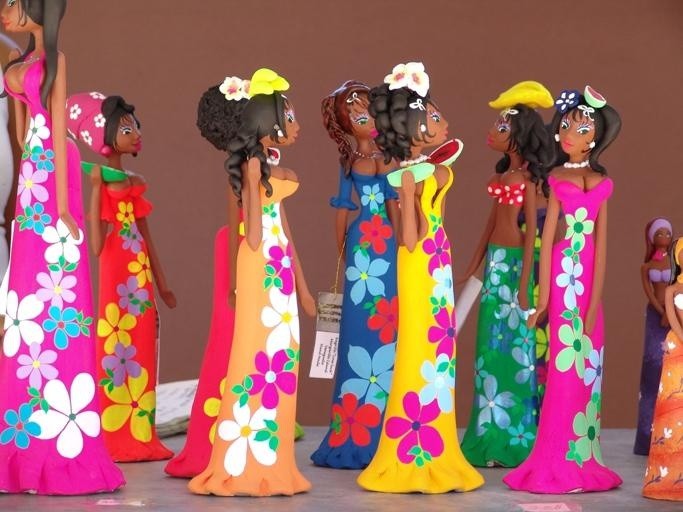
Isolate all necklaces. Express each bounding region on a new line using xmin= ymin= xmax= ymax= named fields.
xmin=267 ymin=147 xmax=281 ymax=165
xmin=355 ymin=148 xmax=378 ymax=159
xmin=399 ymin=155 xmax=430 ymax=167
xmin=564 ymin=159 xmax=589 ymax=168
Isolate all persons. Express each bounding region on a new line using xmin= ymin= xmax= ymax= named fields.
xmin=164 ymin=75 xmax=253 ymax=479
xmin=310 ymin=79 xmax=401 ymax=468
xmin=633 ymin=219 xmax=682 ymax=501
xmin=356 ymin=62 xmax=485 ymax=494
xmin=453 ymin=80 xmax=556 ymax=468
xmin=186 ymin=68 xmax=318 ymax=497
xmin=0 ymin=0 xmax=126 ymax=497
xmin=502 ymin=85 xmax=623 ymax=493
xmin=63 ymin=91 xmax=174 ymax=464
xmin=504 ymin=167 xmax=524 ymax=176
